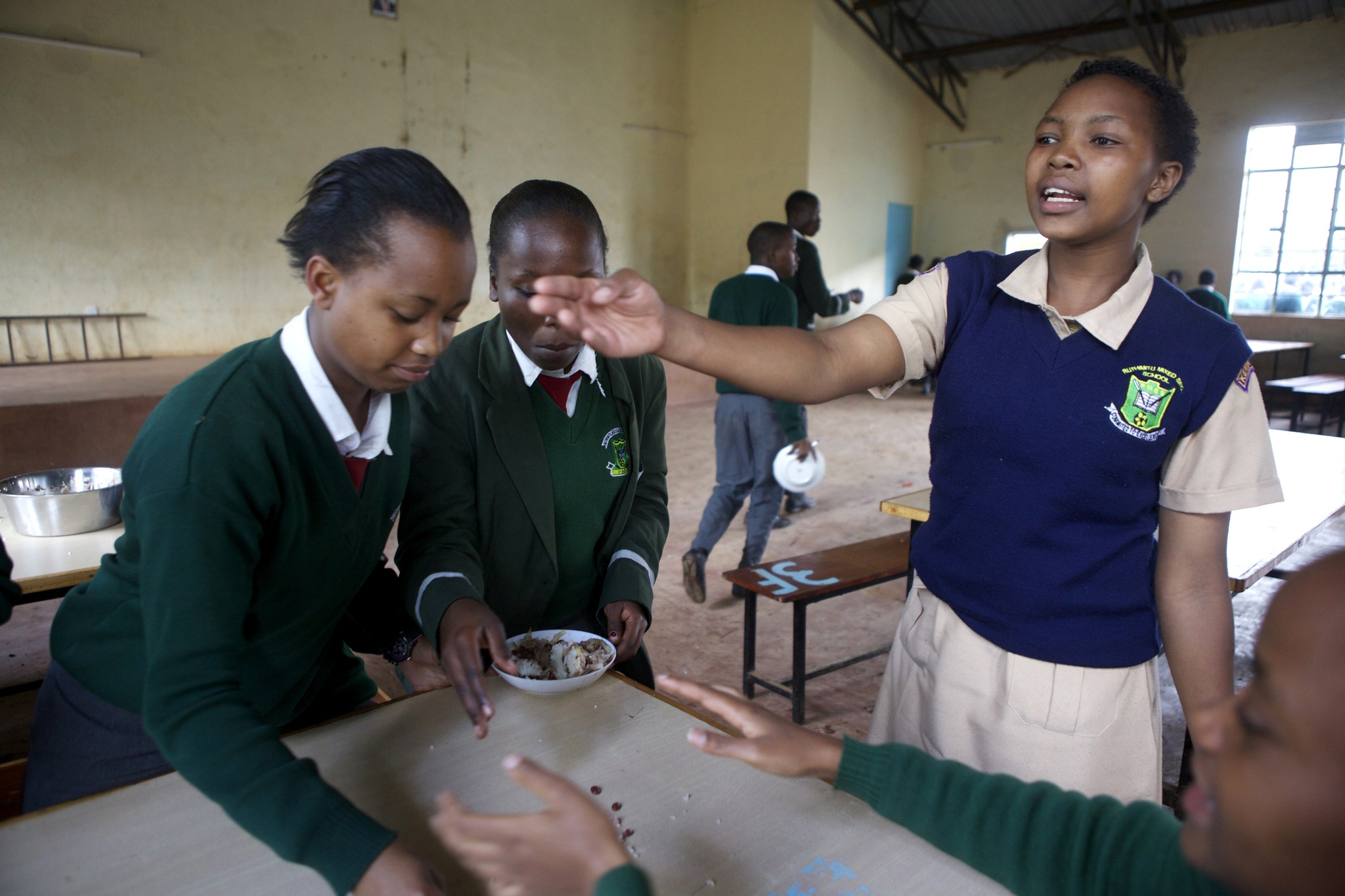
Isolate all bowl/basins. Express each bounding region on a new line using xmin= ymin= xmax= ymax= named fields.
xmin=0 ymin=467 xmax=123 ymax=537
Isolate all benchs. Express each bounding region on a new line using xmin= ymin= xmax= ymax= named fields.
xmin=1264 ymin=374 xmax=1345 ymax=437
xmin=721 ymin=530 xmax=911 ymax=726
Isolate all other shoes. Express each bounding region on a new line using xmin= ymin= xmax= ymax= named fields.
xmin=744 ymin=511 xmax=790 ymax=528
xmin=732 ymin=583 xmax=747 ymax=598
xmin=681 ymin=548 xmax=707 ymax=604
xmin=785 ymin=494 xmax=815 ymax=513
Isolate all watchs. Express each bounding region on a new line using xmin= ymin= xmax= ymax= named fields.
xmin=384 ymin=624 xmax=423 ymax=666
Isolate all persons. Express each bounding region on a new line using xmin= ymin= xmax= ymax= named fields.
xmin=679 ymin=188 xmax=1345 ymax=604
xmin=526 ymin=56 xmax=1285 ymax=820
xmin=435 ymin=457 xmax=1345 ymax=896
xmin=27 ymin=146 xmax=462 ymax=896
xmin=392 ymin=180 xmax=670 ymax=689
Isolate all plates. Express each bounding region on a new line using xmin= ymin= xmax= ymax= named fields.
xmin=773 ymin=443 xmax=826 ymax=492
xmin=491 ymin=629 xmax=616 ymax=695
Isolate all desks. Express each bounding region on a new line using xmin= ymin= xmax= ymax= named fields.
xmin=0 ymin=671 xmax=1012 ymax=896
xmin=880 ymin=428 xmax=1345 ymax=600
xmin=1245 ymin=340 xmax=1315 ymax=422
xmin=0 ymin=517 xmax=126 ymax=606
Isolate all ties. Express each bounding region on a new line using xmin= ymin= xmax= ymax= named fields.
xmin=536 ymin=370 xmax=583 ymax=414
xmin=339 ymin=455 xmax=369 ymax=491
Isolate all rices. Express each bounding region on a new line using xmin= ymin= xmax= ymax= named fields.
xmin=509 ymin=640 xmax=592 ymax=680
